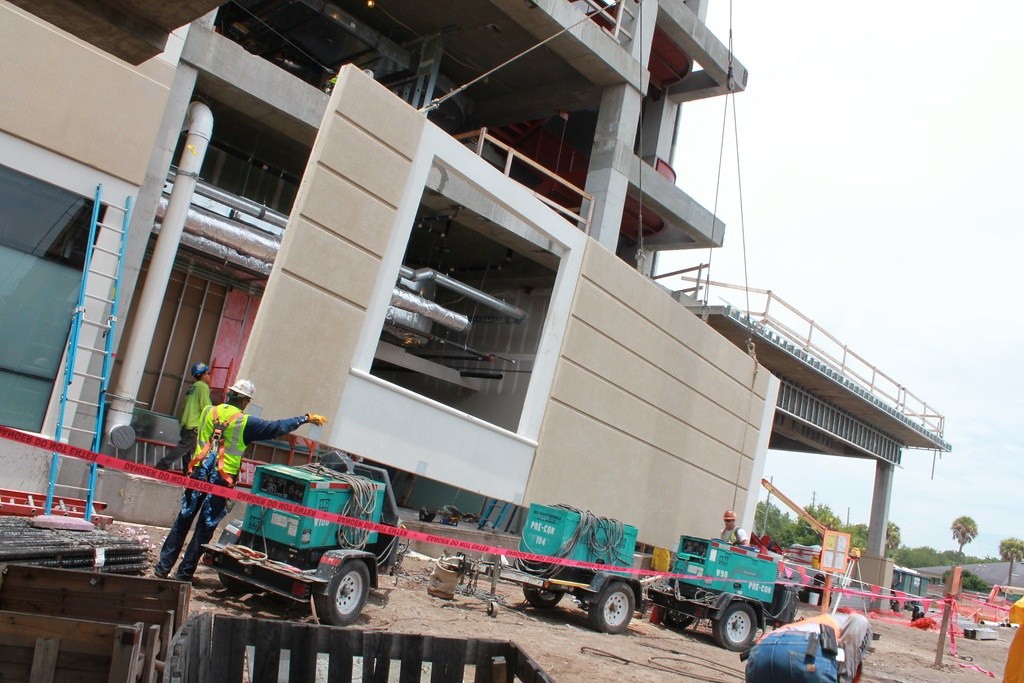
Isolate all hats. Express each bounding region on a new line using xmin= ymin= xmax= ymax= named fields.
xmin=191 ymin=361 xmax=210 ymax=376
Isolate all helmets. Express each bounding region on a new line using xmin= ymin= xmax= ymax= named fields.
xmin=228 ymin=379 xmax=255 ymax=399
xmin=724 ymin=511 xmax=736 ymax=520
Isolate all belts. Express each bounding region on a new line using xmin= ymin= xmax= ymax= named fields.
xmin=773 ymin=632 xmax=822 ymax=637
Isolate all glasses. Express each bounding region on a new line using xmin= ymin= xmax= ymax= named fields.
xmin=207 ymin=372 xmax=211 ymax=375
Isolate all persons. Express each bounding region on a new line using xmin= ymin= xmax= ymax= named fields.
xmin=153 ymin=379 xmax=328 ymax=582
xmin=745 ymin=614 xmax=871 ymax=683
xmin=719 ymin=511 xmax=748 ymax=547
xmin=154 ymin=362 xmax=213 ymax=472
xmin=323 ymin=68 xmax=375 ymax=96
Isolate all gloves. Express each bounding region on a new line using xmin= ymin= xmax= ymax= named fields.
xmin=305 ymin=413 xmax=329 ymax=427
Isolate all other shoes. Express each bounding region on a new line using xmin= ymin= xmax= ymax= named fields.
xmin=191 ymin=576 xmax=200 ymax=585
xmin=149 ymin=573 xmax=164 ymax=579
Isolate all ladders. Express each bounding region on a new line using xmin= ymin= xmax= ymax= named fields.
xmin=43 ymin=181 xmax=135 ymax=519
